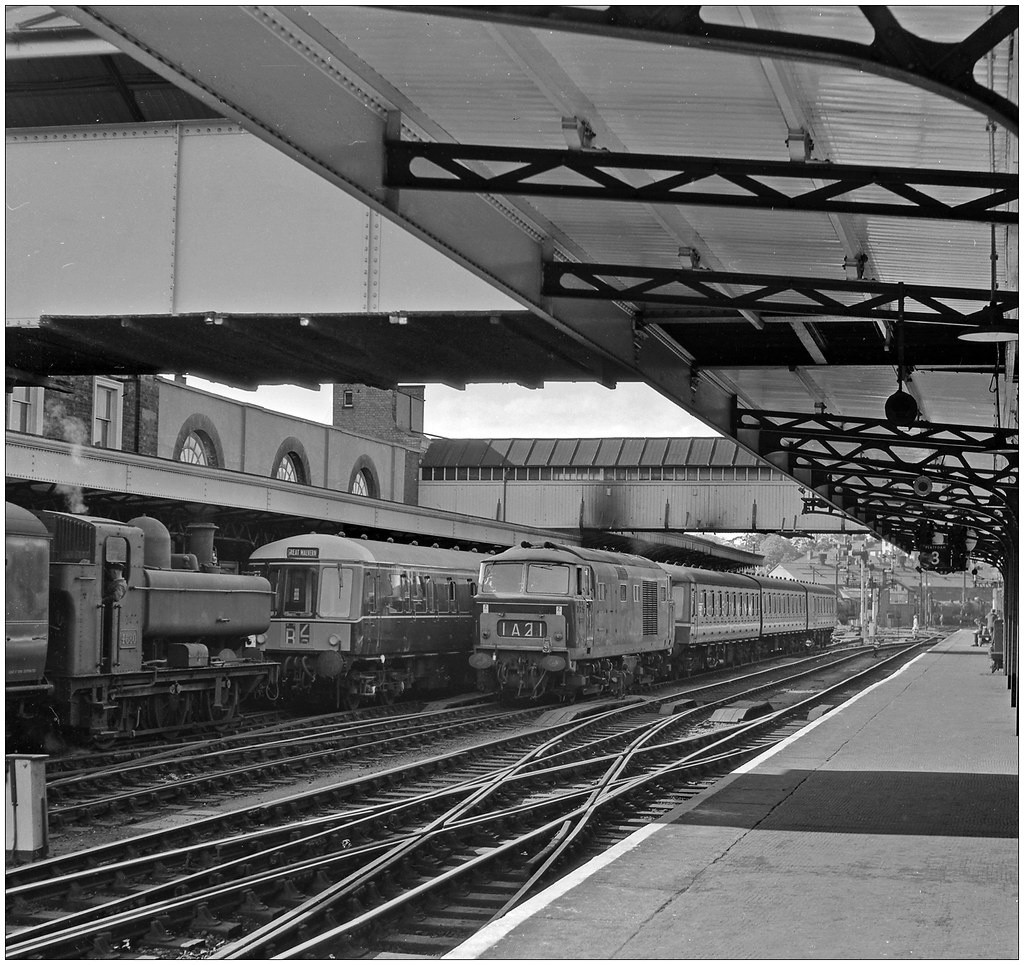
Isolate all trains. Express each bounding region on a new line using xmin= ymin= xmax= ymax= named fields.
xmin=472 ymin=537 xmax=838 ymax=712
xmin=4 ymin=492 xmax=509 ymax=761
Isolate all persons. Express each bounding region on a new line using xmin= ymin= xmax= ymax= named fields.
xmin=972 ymin=609 xmax=999 ymax=645
xmin=910 ymin=613 xmax=919 ymax=637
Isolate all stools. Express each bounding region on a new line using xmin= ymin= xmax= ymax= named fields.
xmin=990 ymin=652 xmax=1003 ymax=673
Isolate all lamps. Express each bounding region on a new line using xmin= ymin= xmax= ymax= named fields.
xmin=913 ymin=475 xmax=933 ymax=496
xmin=954 ymin=223 xmax=1019 ymax=342
xmin=883 ymin=321 xmax=918 ymax=426
